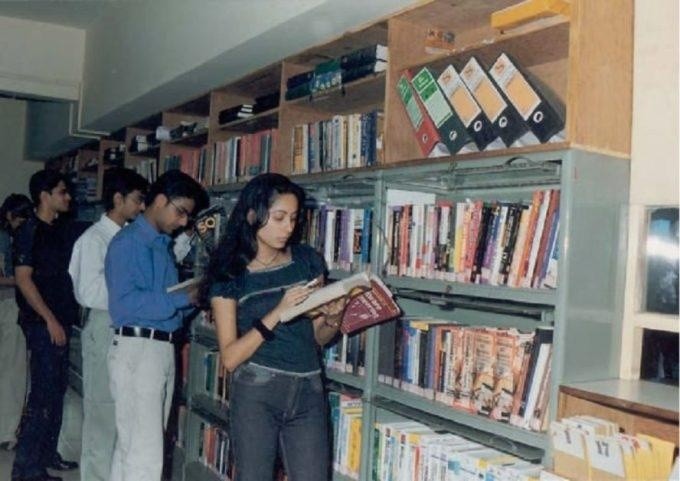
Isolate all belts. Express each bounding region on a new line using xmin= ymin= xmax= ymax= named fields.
xmin=116 ymin=326 xmax=176 ymax=341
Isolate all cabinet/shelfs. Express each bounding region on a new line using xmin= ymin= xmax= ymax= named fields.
xmin=43 ymin=1 xmax=633 ymax=480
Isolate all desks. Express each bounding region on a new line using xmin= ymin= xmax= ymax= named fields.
xmin=556 ymin=378 xmax=679 ymax=481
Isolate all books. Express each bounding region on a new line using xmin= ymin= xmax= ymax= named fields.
xmin=60 ymin=144 xmax=99 ymax=197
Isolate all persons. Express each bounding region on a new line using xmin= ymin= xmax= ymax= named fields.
xmin=11 ymin=167 xmax=81 ymax=481
xmin=67 ymin=166 xmax=148 ymax=481
xmin=0 ymin=192 xmax=37 ymax=451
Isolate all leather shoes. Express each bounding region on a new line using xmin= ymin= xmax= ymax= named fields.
xmin=13 ymin=456 xmax=78 ymax=481
